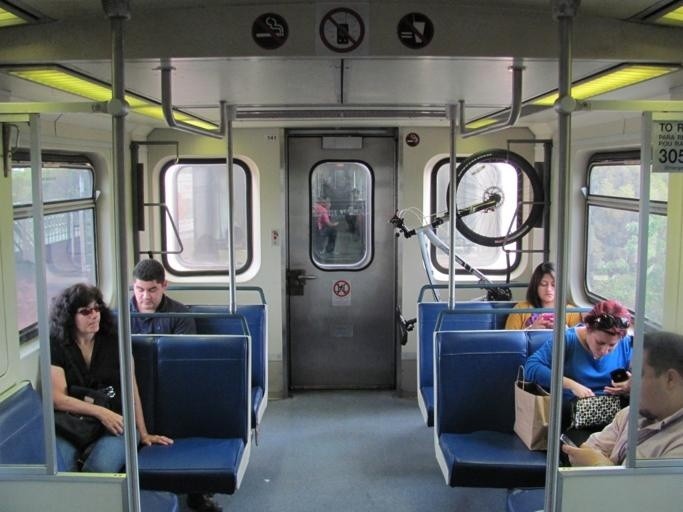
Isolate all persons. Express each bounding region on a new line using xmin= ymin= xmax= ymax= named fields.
xmin=502 ymin=259 xmax=584 ymax=331
xmin=342 ymin=187 xmax=364 ymax=232
xmin=39 ymin=283 xmax=174 ymax=471
xmin=524 ymin=298 xmax=636 ymax=463
xmin=559 ymin=331 xmax=683 ymax=469
xmin=101 ymin=259 xmax=223 ymax=511
xmin=312 ymin=194 xmax=340 ymax=256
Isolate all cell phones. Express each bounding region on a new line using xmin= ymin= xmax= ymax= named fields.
xmin=610 ymin=368 xmax=629 ymax=383
xmin=542 ymin=313 xmax=554 ymax=320
xmin=559 ymin=433 xmax=577 ymax=448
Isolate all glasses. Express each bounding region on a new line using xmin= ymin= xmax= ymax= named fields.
xmin=592 ymin=312 xmax=631 ymax=329
xmin=76 ymin=306 xmax=103 ymax=315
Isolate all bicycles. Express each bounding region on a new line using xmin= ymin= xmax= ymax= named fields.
xmin=381 ymin=140 xmax=541 ymax=345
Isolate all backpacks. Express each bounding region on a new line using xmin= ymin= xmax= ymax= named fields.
xmin=55 ymin=384 xmax=115 ymax=467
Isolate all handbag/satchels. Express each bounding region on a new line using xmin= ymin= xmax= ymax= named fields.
xmin=513 ymin=365 xmax=551 ymax=451
xmin=568 ymin=394 xmax=622 ymax=431
